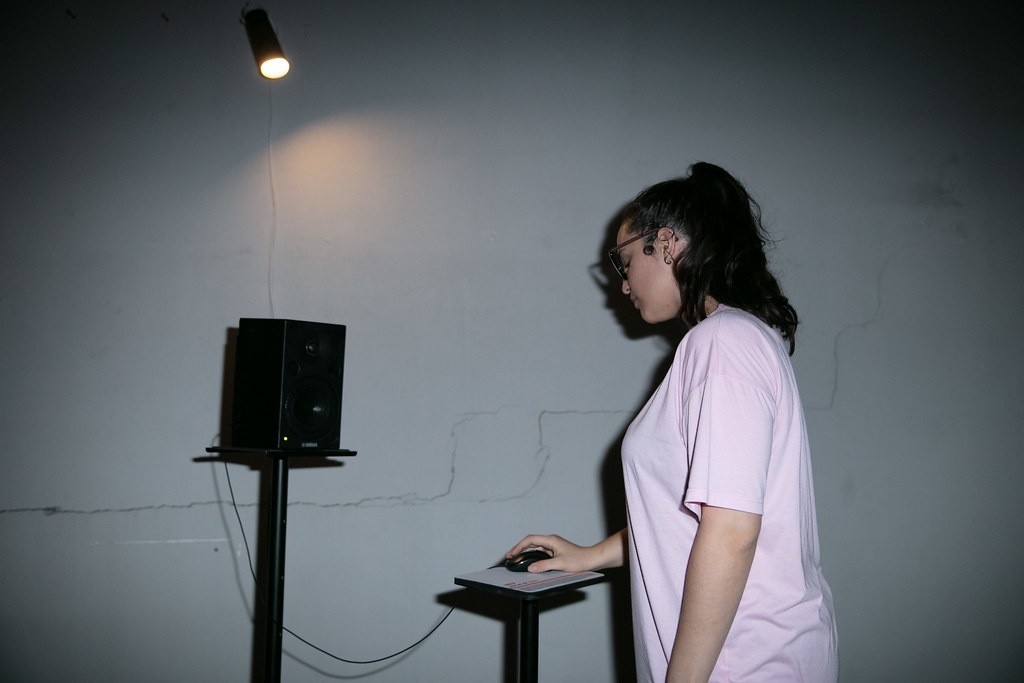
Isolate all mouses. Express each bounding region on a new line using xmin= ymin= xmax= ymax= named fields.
xmin=505 ymin=549 xmax=552 ymax=573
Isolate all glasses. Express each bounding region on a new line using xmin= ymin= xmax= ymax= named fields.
xmin=607 ymin=226 xmax=665 ymax=280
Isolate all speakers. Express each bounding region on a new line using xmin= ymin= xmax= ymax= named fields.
xmin=221 ymin=318 xmax=347 ymax=448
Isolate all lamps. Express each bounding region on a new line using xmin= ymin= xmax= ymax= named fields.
xmin=243 ymin=9 xmax=290 ymax=81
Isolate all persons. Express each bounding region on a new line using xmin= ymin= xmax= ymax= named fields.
xmin=505 ymin=163 xmax=837 ymax=683
